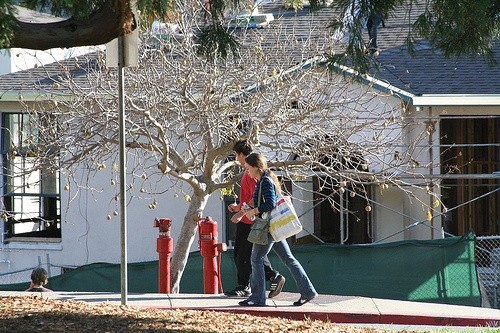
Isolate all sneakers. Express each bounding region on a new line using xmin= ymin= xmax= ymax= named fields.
xmin=239 ymin=299 xmax=257 ymax=306
xmin=268 ymin=274 xmax=286 ymax=298
xmin=224 ymin=285 xmax=252 ymax=299
xmin=294 ymin=292 xmax=319 ymax=306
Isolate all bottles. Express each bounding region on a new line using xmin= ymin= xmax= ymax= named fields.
xmin=243 ymin=202 xmax=256 ymax=221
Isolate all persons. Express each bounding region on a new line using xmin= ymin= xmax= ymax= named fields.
xmin=23 ymin=268 xmax=53 ymax=292
xmin=238 ymin=152 xmax=318 ymax=306
xmin=224 ymin=141 xmax=285 ymax=298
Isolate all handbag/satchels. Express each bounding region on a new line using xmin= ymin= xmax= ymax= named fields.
xmin=247 ymin=218 xmax=270 ymax=245
xmin=262 ymin=177 xmax=302 ymax=243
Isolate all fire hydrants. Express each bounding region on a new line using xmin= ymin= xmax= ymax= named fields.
xmin=153 ymin=218 xmax=173 ymax=294
xmin=197 ymin=215 xmax=227 ymax=294
